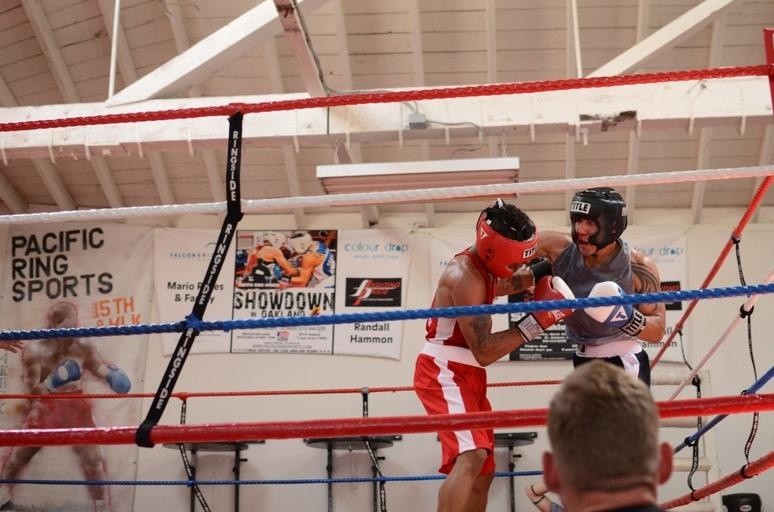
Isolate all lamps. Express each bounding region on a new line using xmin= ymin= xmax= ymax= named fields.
xmin=314 ymin=156 xmax=521 ymax=197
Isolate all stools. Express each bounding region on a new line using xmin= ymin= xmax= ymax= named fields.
xmin=165 ymin=438 xmax=265 ymax=512
xmin=489 ymin=433 xmax=538 ymax=512
xmin=301 ymin=434 xmax=404 ymax=512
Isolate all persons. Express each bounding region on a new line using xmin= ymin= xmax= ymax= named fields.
xmin=540 ymin=357 xmax=676 ymax=511
xmin=0 ymin=300 xmax=133 ymax=511
xmin=409 ymin=197 xmax=579 ymax=511
xmin=234 ymin=231 xmax=335 ymax=289
xmin=531 ymin=184 xmax=667 ymax=388
xmin=523 ymin=480 xmax=565 ymax=511
xmin=0 ymin=327 xmax=23 ymax=355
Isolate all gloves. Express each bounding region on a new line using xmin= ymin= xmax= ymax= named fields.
xmin=514 ymin=274 xmax=575 ymax=346
xmin=97 ymin=359 xmax=131 ymax=394
xmin=42 ymin=356 xmax=82 ymax=392
xmin=582 ymin=279 xmax=648 ymax=339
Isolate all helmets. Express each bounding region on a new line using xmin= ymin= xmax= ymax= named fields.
xmin=475 ymin=198 xmax=541 ymax=281
xmin=289 ymin=230 xmax=311 ymax=254
xmin=262 ymin=231 xmax=285 ymax=249
xmin=568 ymin=185 xmax=626 ymax=248
xmin=45 ymin=301 xmax=77 ymax=330
